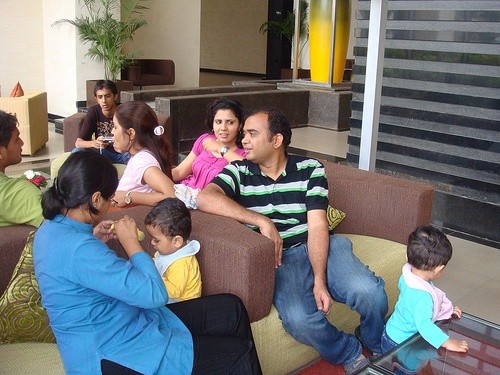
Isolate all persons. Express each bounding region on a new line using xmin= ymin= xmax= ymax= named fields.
xmin=380 ymin=225 xmax=469 ymax=357
xmin=196 ymin=105 xmax=388 ymax=375
xmin=71 ymin=80 xmax=130 ymax=164
xmin=110 ymin=101 xmax=176 ymax=206
xmin=33 ymin=149 xmax=263 ymax=375
xmin=0 ymin=110 xmax=44 ymax=228
xmin=145 ymin=198 xmax=202 ymax=305
xmin=392 ymin=321 xmax=452 ymax=375
xmin=169 ymin=98 xmax=247 ymax=192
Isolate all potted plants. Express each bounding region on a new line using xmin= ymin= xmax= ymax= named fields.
xmin=50 ymin=0 xmax=150 ymax=110
xmin=259 ymin=0 xmax=311 ymax=79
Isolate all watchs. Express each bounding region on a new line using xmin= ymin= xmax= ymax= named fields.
xmin=125 ymin=190 xmax=134 ymax=204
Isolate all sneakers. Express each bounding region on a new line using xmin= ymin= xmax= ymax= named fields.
xmin=356 ymin=325 xmax=383 ymax=360
xmin=344 ymin=354 xmax=370 ymax=375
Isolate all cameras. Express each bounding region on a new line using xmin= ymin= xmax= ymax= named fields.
xmin=104 ymin=136 xmax=114 ymax=143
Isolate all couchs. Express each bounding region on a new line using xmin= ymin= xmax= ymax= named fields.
xmin=121 ymin=59 xmax=175 ymax=90
xmin=0 ymin=85 xmax=48 ymax=156
xmin=64 ymin=112 xmax=179 ymax=166
xmin=0 ymin=153 xmax=433 ymax=375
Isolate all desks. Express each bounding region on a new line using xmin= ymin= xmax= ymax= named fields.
xmin=346 ymin=309 xmax=500 ymax=375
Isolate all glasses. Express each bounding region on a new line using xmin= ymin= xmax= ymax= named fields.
xmin=111 ymin=197 xmax=119 ymax=208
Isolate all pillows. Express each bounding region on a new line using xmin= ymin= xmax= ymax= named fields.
xmin=0 ymin=231 xmax=57 ymax=345
xmin=9 ymin=81 xmax=24 ymax=97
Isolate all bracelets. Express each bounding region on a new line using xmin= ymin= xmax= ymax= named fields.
xmin=220 ymin=146 xmax=229 ymax=156
